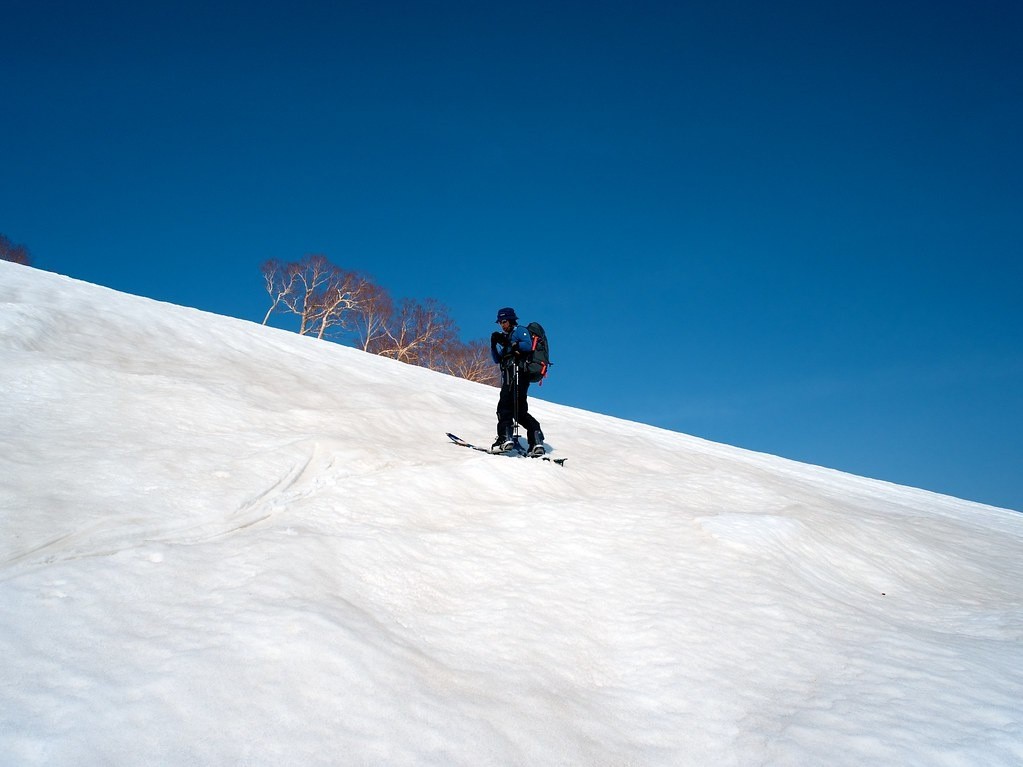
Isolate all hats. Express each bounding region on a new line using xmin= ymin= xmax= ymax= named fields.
xmin=495 ymin=308 xmax=519 ymax=323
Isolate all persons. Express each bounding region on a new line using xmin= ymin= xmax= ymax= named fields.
xmin=491 ymin=308 xmax=546 ymax=456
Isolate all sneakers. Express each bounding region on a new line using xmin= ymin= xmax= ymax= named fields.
xmin=527 ymin=441 xmax=546 ymax=457
xmin=491 ymin=435 xmax=514 ymax=453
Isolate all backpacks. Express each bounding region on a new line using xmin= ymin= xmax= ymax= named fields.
xmin=509 ymin=322 xmax=553 ymax=386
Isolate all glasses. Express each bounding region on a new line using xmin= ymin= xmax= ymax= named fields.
xmin=498 ymin=319 xmax=507 ymax=325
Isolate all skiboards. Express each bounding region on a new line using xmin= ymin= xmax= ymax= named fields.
xmin=445 ymin=431 xmax=569 ymax=468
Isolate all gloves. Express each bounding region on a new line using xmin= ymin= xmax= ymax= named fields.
xmin=491 ymin=332 xmax=508 ymax=348
